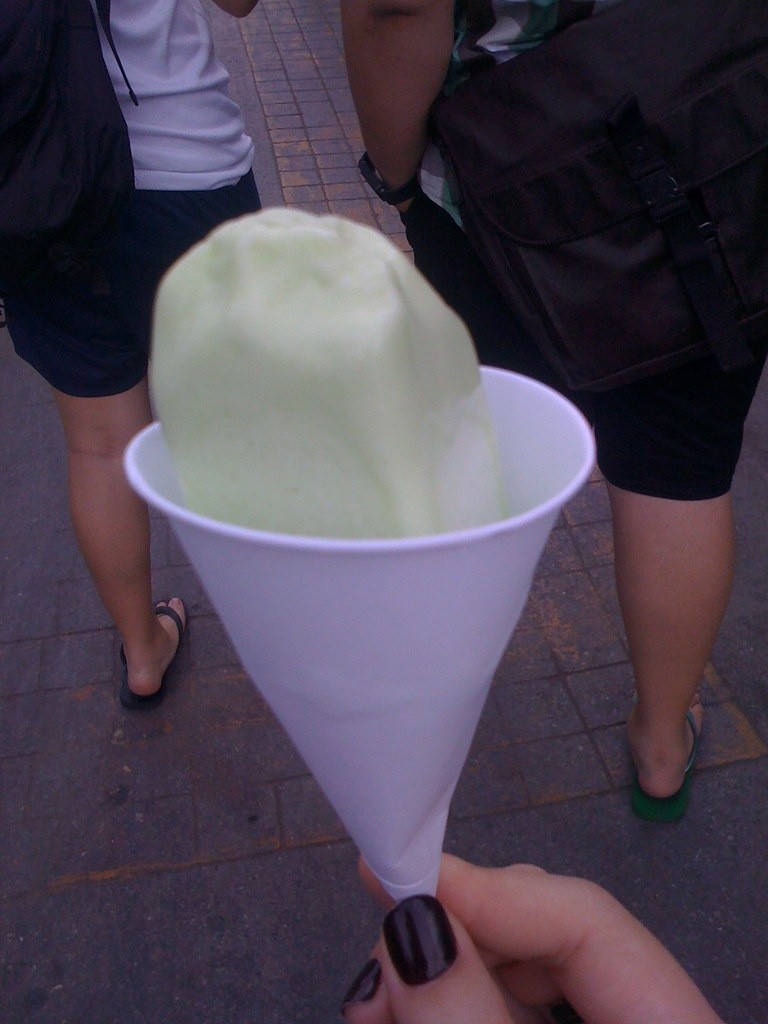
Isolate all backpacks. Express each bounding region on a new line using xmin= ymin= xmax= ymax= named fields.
xmin=0 ymin=0 xmax=132 ymax=251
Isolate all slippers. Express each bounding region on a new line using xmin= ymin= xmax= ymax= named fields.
xmin=631 ymin=693 xmax=701 ymax=823
xmin=119 ymin=600 xmax=184 ymax=713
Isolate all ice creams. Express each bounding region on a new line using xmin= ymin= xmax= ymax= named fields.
xmin=148 ymin=206 xmax=515 ymax=540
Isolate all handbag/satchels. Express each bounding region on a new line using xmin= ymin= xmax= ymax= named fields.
xmin=430 ymin=0 xmax=768 ymax=393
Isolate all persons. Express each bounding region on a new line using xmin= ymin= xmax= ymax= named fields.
xmin=0 ymin=0 xmax=260 ymax=713
xmin=337 ymin=843 xmax=728 ymax=1024
xmin=333 ymin=0 xmax=768 ymax=828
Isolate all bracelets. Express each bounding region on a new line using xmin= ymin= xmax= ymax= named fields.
xmin=357 ymin=147 xmax=423 ymax=206
